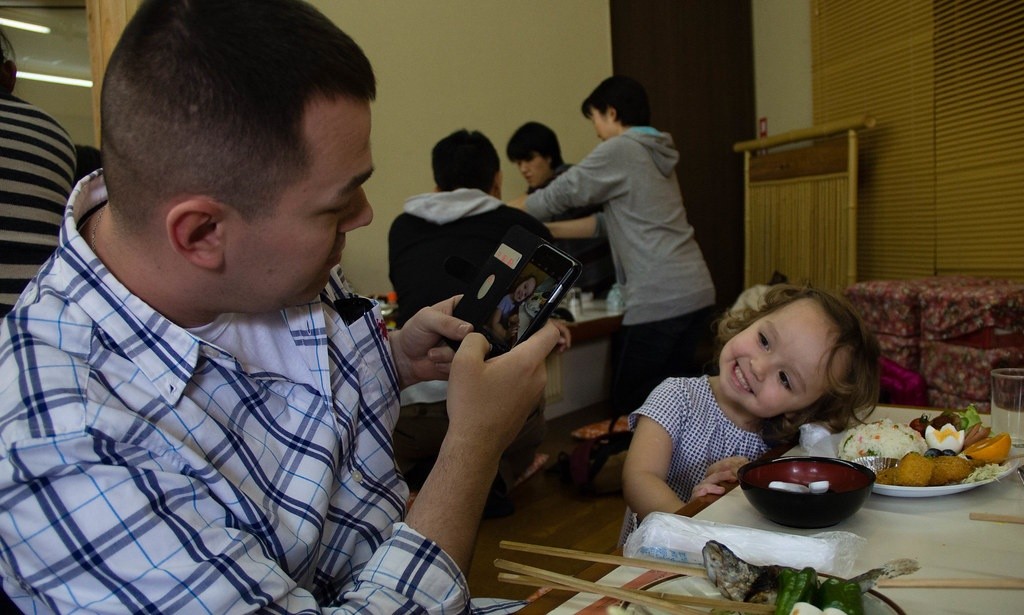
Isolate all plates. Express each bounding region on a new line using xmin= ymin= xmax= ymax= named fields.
xmin=615 ymin=571 xmax=906 ymax=615
xmin=872 ymin=445 xmax=1021 ymax=499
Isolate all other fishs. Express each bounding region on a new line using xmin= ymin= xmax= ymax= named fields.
xmin=702 ymin=539 xmax=922 ymax=605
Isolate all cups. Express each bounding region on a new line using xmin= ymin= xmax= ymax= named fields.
xmin=990 ymin=369 xmax=1022 ymax=459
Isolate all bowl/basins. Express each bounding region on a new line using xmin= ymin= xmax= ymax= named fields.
xmin=738 ymin=456 xmax=876 ymax=529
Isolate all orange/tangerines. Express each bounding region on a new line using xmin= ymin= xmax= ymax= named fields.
xmin=961 ymin=433 xmax=1011 ymax=464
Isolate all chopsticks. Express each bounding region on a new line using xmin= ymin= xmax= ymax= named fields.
xmin=491 ymin=557 xmax=772 ymax=615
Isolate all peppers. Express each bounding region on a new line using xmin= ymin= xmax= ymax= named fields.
xmin=774 ymin=566 xmax=865 ymax=615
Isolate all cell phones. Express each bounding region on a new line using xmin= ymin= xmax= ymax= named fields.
xmin=478 ymin=244 xmax=576 ymax=364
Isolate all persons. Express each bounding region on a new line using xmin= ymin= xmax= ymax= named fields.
xmin=489 ymin=275 xmax=539 ymax=342
xmin=504 ymin=75 xmax=717 ymax=420
xmin=617 ymin=284 xmax=883 ymax=554
xmin=72 ymin=142 xmax=103 ymax=182
xmin=387 ymin=127 xmax=561 ymax=497
xmin=1 ymin=24 xmax=78 ymax=326
xmin=0 ymin=1 xmax=574 ymax=615
xmin=497 ymin=121 xmax=619 ymax=301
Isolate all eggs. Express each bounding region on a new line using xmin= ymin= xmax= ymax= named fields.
xmin=923 ymin=423 xmax=964 ymax=456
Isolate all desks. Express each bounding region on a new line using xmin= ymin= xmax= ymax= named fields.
xmin=532 ymin=290 xmax=620 ymax=443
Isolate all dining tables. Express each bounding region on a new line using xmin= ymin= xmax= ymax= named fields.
xmin=504 ymin=405 xmax=1022 ymax=614
